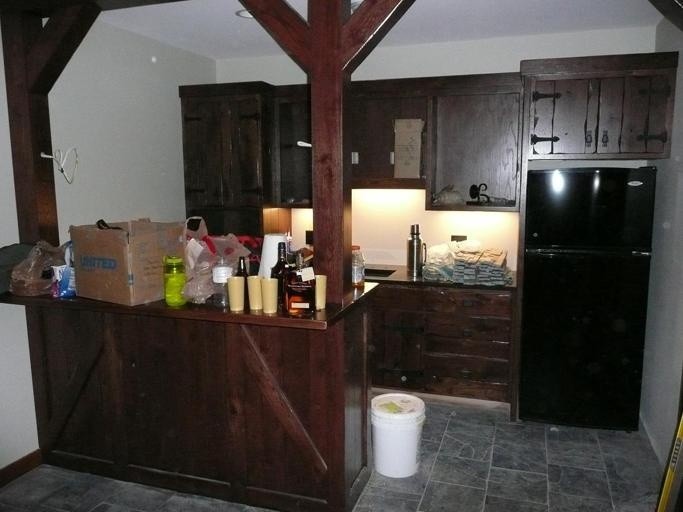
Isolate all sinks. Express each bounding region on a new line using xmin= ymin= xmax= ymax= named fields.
xmin=364 ymin=267 xmax=396 ymax=278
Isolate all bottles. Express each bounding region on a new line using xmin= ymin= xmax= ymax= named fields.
xmin=283 ymin=253 xmax=316 ymax=319
xmin=351 ymin=245 xmax=365 ymax=289
xmin=211 ymin=257 xmax=235 ymax=309
xmin=162 ymin=255 xmax=188 ymax=307
xmin=271 ymin=242 xmax=292 ymax=307
xmin=235 ymin=256 xmax=249 ymax=308
xmin=406 ymin=224 xmax=427 ymax=279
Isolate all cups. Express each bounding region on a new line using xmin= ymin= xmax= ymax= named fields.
xmin=314 ymin=274 xmax=328 ymax=311
xmin=227 ymin=276 xmax=245 ymax=312
xmin=260 ymin=277 xmax=278 ymax=314
xmin=246 ymin=276 xmax=265 ymax=313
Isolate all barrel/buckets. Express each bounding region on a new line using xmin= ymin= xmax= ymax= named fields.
xmin=371 ymin=393 xmax=426 ymax=478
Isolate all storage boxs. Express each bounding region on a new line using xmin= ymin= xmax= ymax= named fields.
xmin=68 ymin=216 xmax=184 ymax=307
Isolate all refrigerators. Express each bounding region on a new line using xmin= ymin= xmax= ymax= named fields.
xmin=517 ymin=166 xmax=657 ymax=434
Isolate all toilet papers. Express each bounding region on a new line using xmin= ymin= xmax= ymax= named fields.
xmin=258 ymin=233 xmax=287 ymax=279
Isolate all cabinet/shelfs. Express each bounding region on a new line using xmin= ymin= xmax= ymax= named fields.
xmin=518 ymin=50 xmax=679 ymax=161
xmin=178 ymin=81 xmax=291 ymax=235
xmin=367 ymin=282 xmax=513 ymax=403
xmin=270 ymin=72 xmax=522 ymax=213
